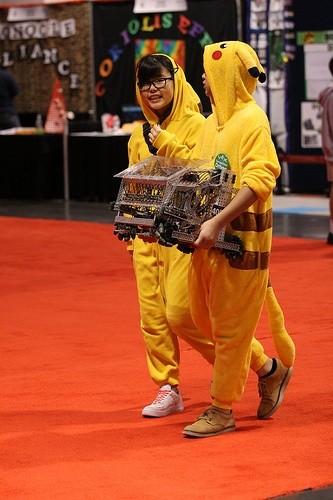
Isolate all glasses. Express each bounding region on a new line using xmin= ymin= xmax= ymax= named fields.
xmin=137 ymin=77 xmax=172 ymax=90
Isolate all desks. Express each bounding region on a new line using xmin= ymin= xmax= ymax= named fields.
xmin=0 ymin=127 xmax=127 ymax=203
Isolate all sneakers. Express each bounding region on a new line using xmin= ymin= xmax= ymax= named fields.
xmin=256 ymin=357 xmax=294 ymax=420
xmin=181 ymin=405 xmax=237 ymax=438
xmin=141 ymin=384 xmax=184 ymax=416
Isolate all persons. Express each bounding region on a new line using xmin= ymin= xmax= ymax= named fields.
xmin=318 ymin=57 xmax=333 ymax=244
xmin=110 ymin=40 xmax=291 ymax=437
xmin=0 ymin=56 xmax=22 ymax=128
